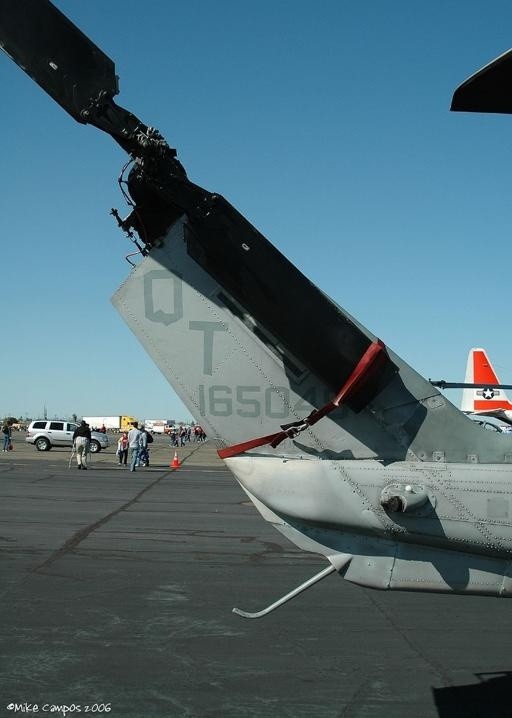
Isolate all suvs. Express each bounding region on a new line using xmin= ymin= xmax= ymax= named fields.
xmin=25 ymin=420 xmax=112 ymax=454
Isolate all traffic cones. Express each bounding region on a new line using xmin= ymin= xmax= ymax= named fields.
xmin=170 ymin=450 xmax=182 ymax=468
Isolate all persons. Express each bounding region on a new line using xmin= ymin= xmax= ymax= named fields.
xmin=3 ymin=420 xmax=12 ymax=452
xmin=139 ymin=425 xmax=153 ymax=466
xmin=117 ymin=432 xmax=128 ymax=466
xmin=91 ymin=424 xmax=107 ymax=433
xmin=72 ymin=421 xmax=91 ymax=470
xmin=167 ymin=426 xmax=204 ymax=446
xmin=128 ymin=422 xmax=141 ymax=472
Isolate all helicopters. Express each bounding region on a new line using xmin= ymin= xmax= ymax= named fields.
xmin=0 ymin=1 xmax=512 ymax=623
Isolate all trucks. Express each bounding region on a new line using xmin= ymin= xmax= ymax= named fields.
xmin=82 ymin=415 xmax=139 ymax=433
xmin=144 ymin=419 xmax=180 ymax=436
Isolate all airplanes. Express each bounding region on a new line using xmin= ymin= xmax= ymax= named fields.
xmin=459 ymin=347 xmax=512 ymax=433
